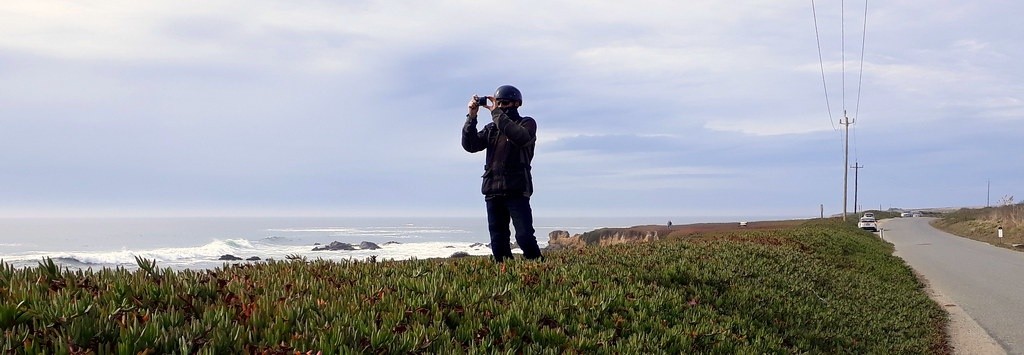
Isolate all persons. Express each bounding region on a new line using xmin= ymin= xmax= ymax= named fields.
xmin=461 ymin=86 xmax=546 ymax=263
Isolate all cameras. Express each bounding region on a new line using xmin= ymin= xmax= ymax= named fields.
xmin=476 ymin=96 xmax=487 ymax=105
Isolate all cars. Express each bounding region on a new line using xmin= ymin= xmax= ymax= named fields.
xmin=904 ymin=211 xmax=912 ymax=216
xmin=858 ymin=212 xmax=878 ymax=231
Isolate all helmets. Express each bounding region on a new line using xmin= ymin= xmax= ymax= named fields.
xmin=494 ymin=85 xmax=523 ymax=106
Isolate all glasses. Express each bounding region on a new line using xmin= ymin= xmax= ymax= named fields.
xmin=496 ymin=101 xmax=512 ymax=105
